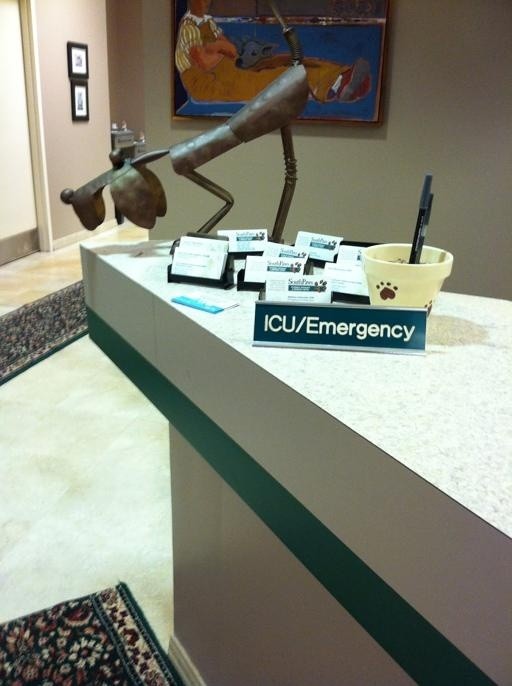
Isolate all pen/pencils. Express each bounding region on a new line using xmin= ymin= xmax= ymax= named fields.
xmin=408 ymin=175 xmax=434 ymax=263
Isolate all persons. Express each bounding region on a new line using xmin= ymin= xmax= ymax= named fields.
xmin=174 ymin=1 xmax=371 ymax=104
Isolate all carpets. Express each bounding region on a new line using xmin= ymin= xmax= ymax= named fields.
xmin=0 ymin=583 xmax=188 ymax=686
xmin=0 ymin=279 xmax=88 ymax=390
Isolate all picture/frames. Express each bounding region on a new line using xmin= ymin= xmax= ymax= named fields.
xmin=67 ymin=40 xmax=89 ymax=124
xmin=171 ymin=1 xmax=390 ymax=128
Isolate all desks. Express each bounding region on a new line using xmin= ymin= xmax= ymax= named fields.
xmin=79 ymin=238 xmax=512 ymax=686
